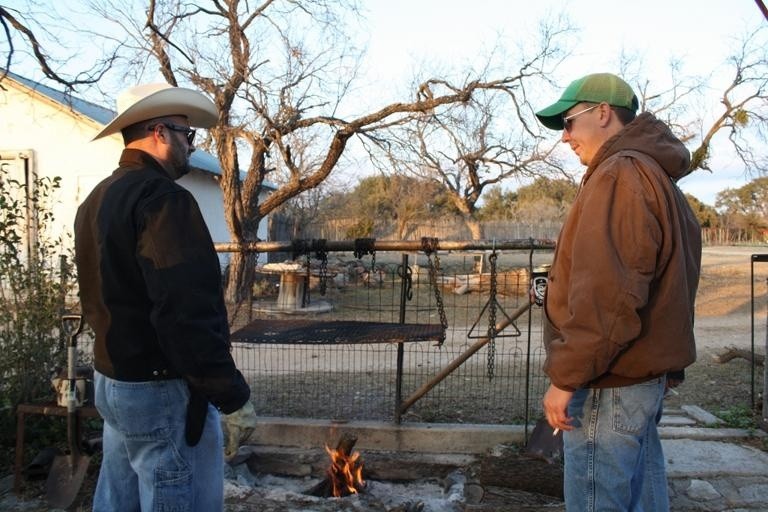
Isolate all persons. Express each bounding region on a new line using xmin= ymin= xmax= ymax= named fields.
xmin=534 ymin=72 xmax=703 ymax=512
xmin=72 ymin=84 xmax=251 ymax=511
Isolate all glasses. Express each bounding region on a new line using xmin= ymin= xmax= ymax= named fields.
xmin=149 ymin=123 xmax=197 ymax=144
xmin=563 ymin=104 xmax=597 ymax=131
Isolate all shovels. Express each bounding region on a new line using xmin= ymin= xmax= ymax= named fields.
xmin=44 ymin=313 xmax=93 ymax=512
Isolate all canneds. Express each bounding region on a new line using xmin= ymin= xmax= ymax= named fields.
xmin=531 ymin=268 xmax=550 ymax=308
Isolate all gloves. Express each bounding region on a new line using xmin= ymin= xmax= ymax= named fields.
xmin=223 ymin=399 xmax=258 ymax=462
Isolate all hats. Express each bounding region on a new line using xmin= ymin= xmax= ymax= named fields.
xmin=90 ymin=82 xmax=219 ymax=142
xmin=535 ymin=74 xmax=639 ymax=130
